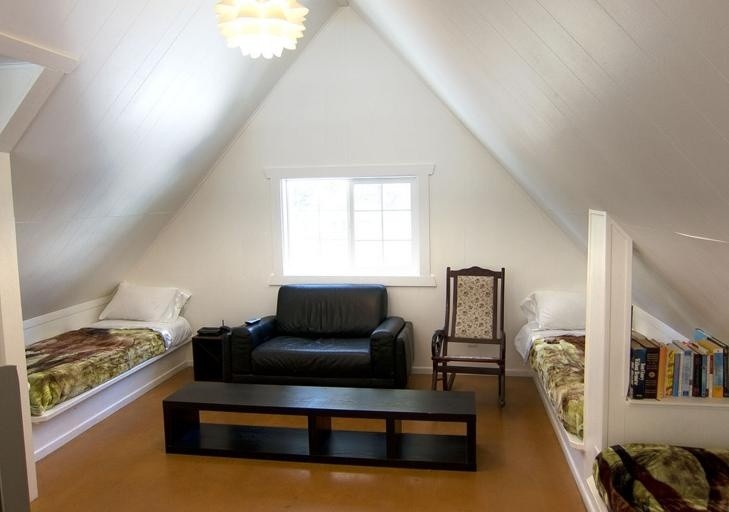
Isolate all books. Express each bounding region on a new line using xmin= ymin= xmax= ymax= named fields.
xmin=627 ymin=326 xmax=729 ymax=402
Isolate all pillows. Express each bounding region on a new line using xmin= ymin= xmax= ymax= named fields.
xmin=520 ymin=289 xmax=587 ymax=330
xmin=99 ymin=280 xmax=192 ymax=322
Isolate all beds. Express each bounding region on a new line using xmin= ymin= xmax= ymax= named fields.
xmin=527 ymin=317 xmax=585 ymax=500
xmin=584 ymin=443 xmax=729 ymax=512
xmin=22 ymin=294 xmax=195 ymax=462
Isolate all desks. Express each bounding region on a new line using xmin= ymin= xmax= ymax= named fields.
xmin=192 ymin=332 xmax=228 ymax=381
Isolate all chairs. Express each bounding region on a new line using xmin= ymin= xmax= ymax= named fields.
xmin=430 ymin=266 xmax=506 ymax=409
xmin=223 ymin=285 xmax=415 ymax=389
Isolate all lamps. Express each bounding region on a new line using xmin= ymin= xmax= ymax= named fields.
xmin=212 ymin=0 xmax=309 ymax=59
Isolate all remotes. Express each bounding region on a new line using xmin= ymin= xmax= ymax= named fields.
xmin=244 ymin=316 xmax=262 ymax=324
